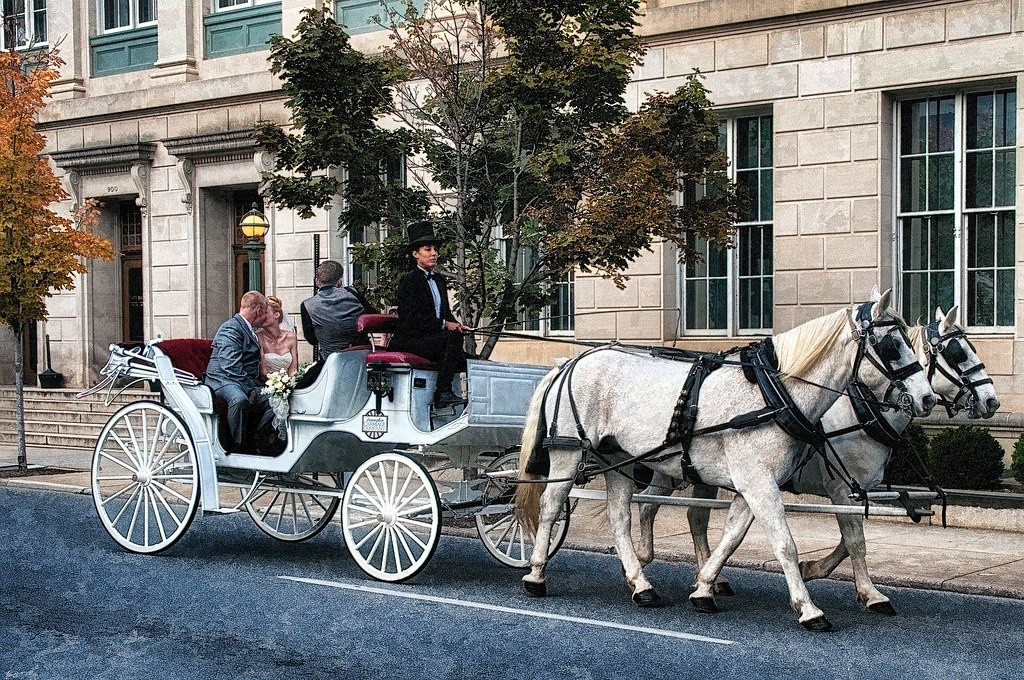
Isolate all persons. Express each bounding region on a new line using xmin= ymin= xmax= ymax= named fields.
xmin=388 ymin=221 xmax=490 ymax=409
xmin=295 ymin=260 xmax=383 ymax=389
xmin=205 ymin=290 xmax=276 ymax=454
xmin=254 ymin=295 xmax=298 ymax=382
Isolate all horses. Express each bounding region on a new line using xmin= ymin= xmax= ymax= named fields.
xmin=506 ymin=286 xmax=940 ymax=632
xmin=619 ymin=304 xmax=1001 ymax=617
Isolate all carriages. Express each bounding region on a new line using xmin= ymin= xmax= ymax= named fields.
xmin=82 ymin=224 xmax=998 ymax=634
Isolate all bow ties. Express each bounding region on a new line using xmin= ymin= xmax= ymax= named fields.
xmin=423 ymin=271 xmax=436 ymax=281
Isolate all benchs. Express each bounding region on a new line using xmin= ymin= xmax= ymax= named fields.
xmin=146 ymin=339 xmax=227 ymax=415
xmin=358 ymin=314 xmax=433 ymax=365
xmin=290 ymin=345 xmax=387 ymax=418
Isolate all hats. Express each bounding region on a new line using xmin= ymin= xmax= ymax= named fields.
xmin=405 ymin=222 xmax=441 ymax=252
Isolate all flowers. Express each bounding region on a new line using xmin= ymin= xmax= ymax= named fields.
xmin=261 ymin=368 xmax=298 ymax=400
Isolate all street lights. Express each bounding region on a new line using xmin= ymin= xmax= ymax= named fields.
xmin=240 ymin=204 xmax=269 ymax=293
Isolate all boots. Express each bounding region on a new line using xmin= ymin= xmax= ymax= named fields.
xmin=435 ymin=362 xmax=463 ymax=403
